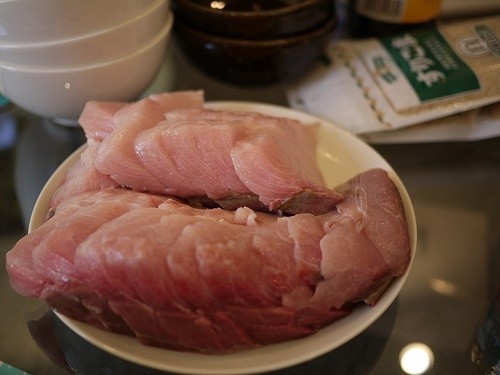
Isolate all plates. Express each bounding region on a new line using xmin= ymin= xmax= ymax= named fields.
xmin=27 ymin=100 xmax=422 ymax=374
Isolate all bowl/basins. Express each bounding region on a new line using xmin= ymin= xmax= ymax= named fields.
xmin=1 ymin=1 xmax=174 ymax=126
xmin=174 ymin=0 xmax=338 ymax=87
